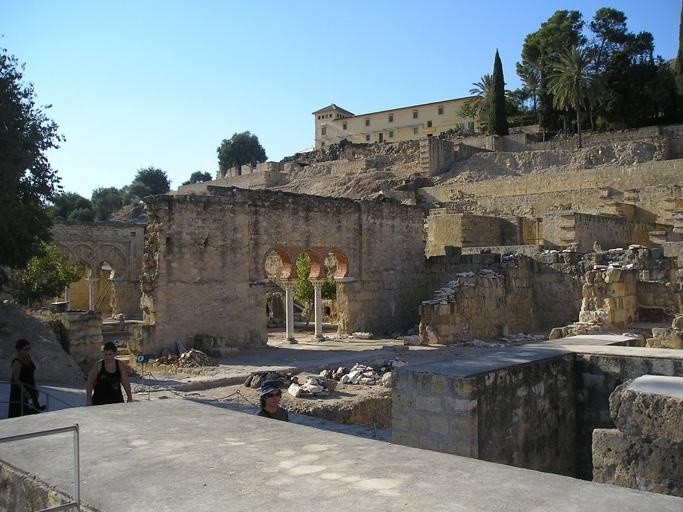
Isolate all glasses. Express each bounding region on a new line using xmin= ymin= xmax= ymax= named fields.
xmin=268 ymin=392 xmax=280 ymax=398
xmin=24 ymin=347 xmax=31 ymax=351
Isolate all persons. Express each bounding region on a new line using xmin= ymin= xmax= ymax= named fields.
xmin=8 ymin=339 xmax=46 ymax=418
xmin=86 ymin=341 xmax=132 ymax=406
xmin=255 ymin=379 xmax=288 ymax=422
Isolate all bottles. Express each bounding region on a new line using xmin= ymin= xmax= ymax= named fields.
xmin=27 ymin=399 xmax=33 ymax=409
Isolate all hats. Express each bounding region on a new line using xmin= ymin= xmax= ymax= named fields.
xmin=259 ymin=380 xmax=280 ymax=398
